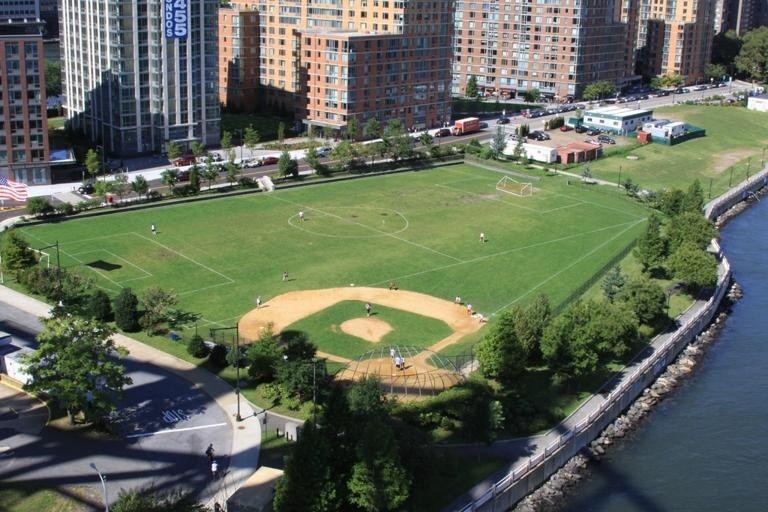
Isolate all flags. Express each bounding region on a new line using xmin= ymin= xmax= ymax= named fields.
xmin=0 ymin=176 xmax=28 ymax=203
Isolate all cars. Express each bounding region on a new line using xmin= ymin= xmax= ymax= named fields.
xmin=497 ymin=119 xmax=509 ymax=124
xmin=617 ymin=83 xmax=765 ymax=103
xmin=435 ymin=129 xmax=450 ymax=137
xmin=174 ymin=145 xmax=334 ymax=182
xmin=527 ymin=130 xmax=549 ymax=141
xmin=527 ymin=104 xmax=585 ymax=118
xmin=79 ymin=183 xmax=96 ymax=195
xmin=560 ymin=125 xmax=615 ymax=145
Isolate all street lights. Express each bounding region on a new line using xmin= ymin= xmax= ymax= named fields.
xmin=283 ymin=355 xmax=316 ymax=425
xmin=96 ymin=144 xmax=107 ymax=203
xmin=208 ymin=322 xmax=241 ymax=421
xmin=38 ymin=239 xmax=60 ymax=268
xmin=89 ymin=462 xmax=109 ymax=512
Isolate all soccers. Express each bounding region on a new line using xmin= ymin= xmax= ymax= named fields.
xmin=349 ymin=283 xmax=354 ymax=286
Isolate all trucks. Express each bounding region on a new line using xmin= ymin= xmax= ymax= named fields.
xmin=452 ymin=116 xmax=479 ymax=136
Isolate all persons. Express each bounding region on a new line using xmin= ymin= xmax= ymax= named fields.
xmin=467 ymin=303 xmax=472 ymax=313
xmin=86 ymin=384 xmax=100 ymax=414
xmin=480 ymin=231 xmax=485 ymax=243
xmin=390 ymin=348 xmax=395 ymax=358
xmin=214 ymin=502 xmax=224 ymax=512
xmin=477 ymin=314 xmax=483 ymax=321
xmin=257 ymin=295 xmax=262 ymax=306
xmin=151 ymin=224 xmax=156 ymax=238
xmin=455 ymin=296 xmax=461 ymax=304
xmin=205 ymin=443 xmax=214 ymax=460
xmin=281 ymin=271 xmax=288 ymax=281
xmin=211 ymin=459 xmax=218 ymax=480
xmin=365 ymin=303 xmax=372 ymax=317
xmin=394 ymin=356 xmax=401 ymax=368
xmin=299 ymin=210 xmax=304 ymax=224
xmin=400 ymin=355 xmax=405 ymax=366
